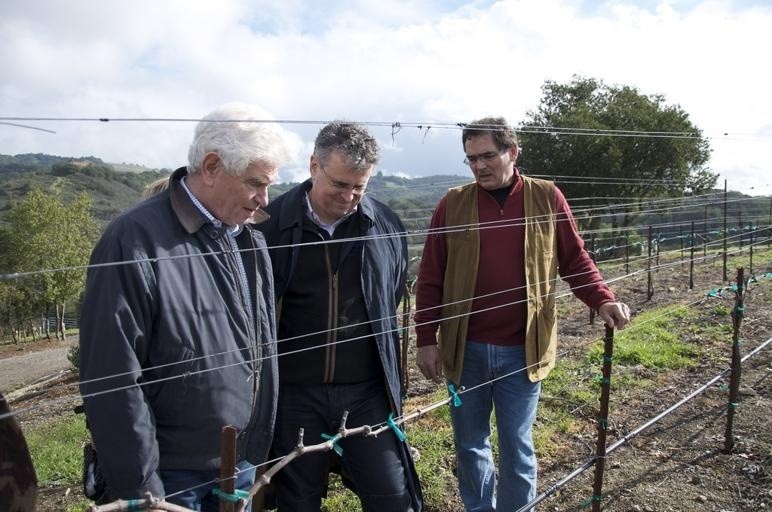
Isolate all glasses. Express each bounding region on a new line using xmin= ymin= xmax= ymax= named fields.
xmin=320 ymin=166 xmax=367 ymax=192
xmin=462 ymin=146 xmax=506 ymax=164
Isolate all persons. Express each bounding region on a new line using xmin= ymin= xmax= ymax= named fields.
xmin=75 ymin=100 xmax=283 ymax=512
xmin=412 ymin=115 xmax=636 ymax=512
xmin=244 ymin=117 xmax=429 ymax=509
